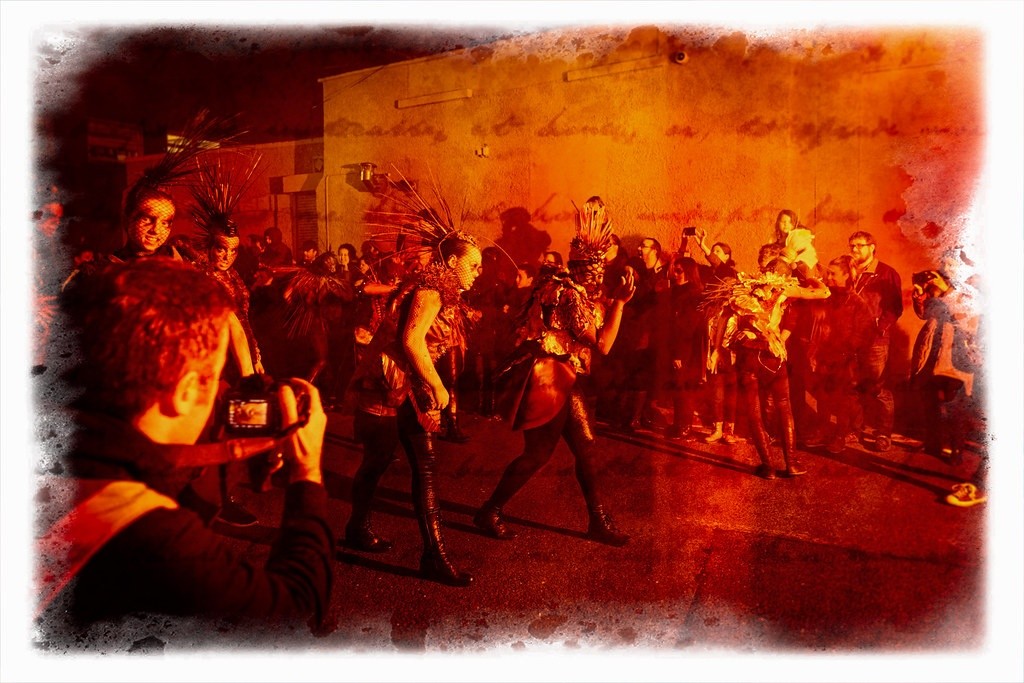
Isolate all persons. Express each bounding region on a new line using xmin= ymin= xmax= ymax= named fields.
xmin=34 ymin=188 xmax=984 ymax=590
xmin=33 ymin=254 xmax=334 ymax=649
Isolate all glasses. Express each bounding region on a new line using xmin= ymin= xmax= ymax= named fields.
xmin=849 ymin=243 xmax=872 ymax=249
xmin=638 ymin=244 xmax=655 ymax=249
xmin=673 ymin=268 xmax=685 ymax=275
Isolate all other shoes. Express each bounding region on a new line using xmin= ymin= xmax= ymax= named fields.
xmin=804 ymin=431 xmax=833 ymax=446
xmin=945 ymin=483 xmax=987 ymax=507
xmin=677 ymin=415 xmax=692 ymax=437
xmin=875 ymin=433 xmax=892 ymax=451
xmin=628 ymin=416 xmax=641 ymax=429
xmin=826 ymin=433 xmax=846 ymax=453
xmin=608 ymin=414 xmax=624 ymax=433
xmin=641 ymin=415 xmax=658 ymax=430
xmin=437 ymin=418 xmax=471 ymax=442
xmin=947 ymin=450 xmax=963 ymax=466
xmin=844 ymin=430 xmax=865 ymax=442
xmin=216 ymin=501 xmax=259 ymax=525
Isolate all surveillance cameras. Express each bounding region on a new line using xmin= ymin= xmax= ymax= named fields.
xmin=675 ymin=50 xmax=688 ymax=64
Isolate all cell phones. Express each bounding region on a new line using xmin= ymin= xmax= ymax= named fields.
xmin=683 ymin=226 xmax=697 ymax=236
xmin=914 ymin=270 xmax=934 ymax=284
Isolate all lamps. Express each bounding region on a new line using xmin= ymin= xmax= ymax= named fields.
xmin=359 ymin=162 xmax=379 ymax=191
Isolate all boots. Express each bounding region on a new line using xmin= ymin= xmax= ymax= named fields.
xmin=413 ymin=508 xmax=474 ymax=585
xmin=753 ymin=431 xmax=776 ymax=480
xmin=344 ymin=486 xmax=393 ymax=550
xmin=724 ymin=422 xmax=735 ymax=443
xmin=704 ymin=421 xmax=723 ymax=442
xmin=587 ymin=505 xmax=631 ymax=545
xmin=477 ymin=378 xmax=497 ymax=416
xmin=784 ymin=427 xmax=807 ymax=475
xmin=474 ymin=500 xmax=519 ymax=540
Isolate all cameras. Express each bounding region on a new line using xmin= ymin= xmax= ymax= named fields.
xmin=222 ymin=384 xmax=311 ymax=436
